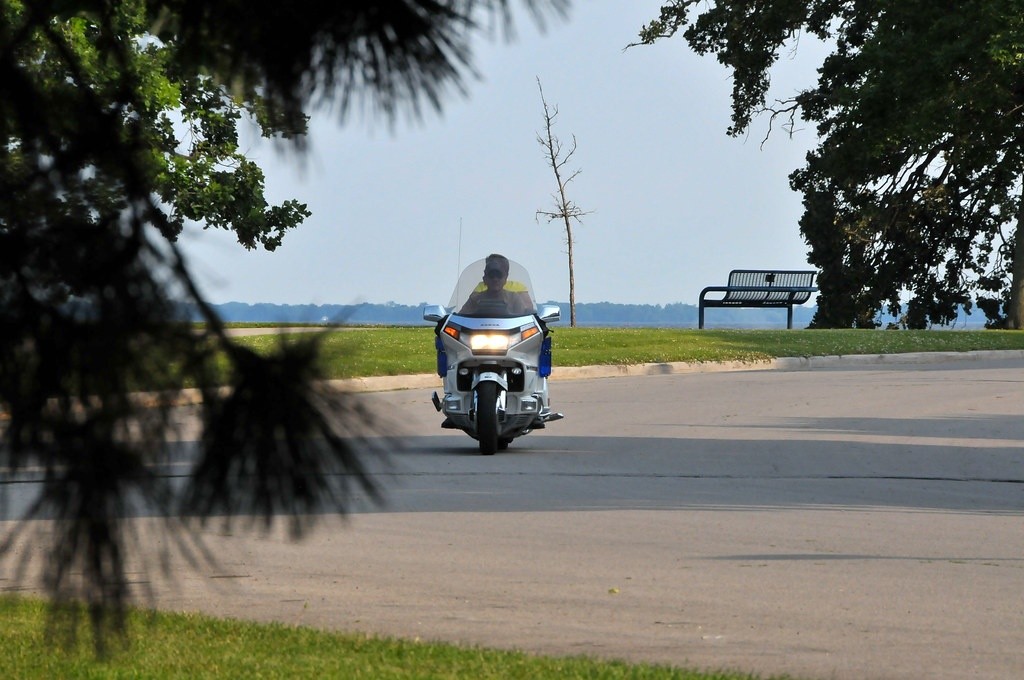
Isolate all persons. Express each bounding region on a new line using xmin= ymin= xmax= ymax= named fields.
xmin=458 ymin=254 xmax=533 ymax=317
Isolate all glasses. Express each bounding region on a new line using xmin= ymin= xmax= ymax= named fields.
xmin=489 ymin=271 xmax=503 ymax=278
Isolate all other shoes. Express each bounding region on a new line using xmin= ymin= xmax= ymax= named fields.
xmin=529 ymin=419 xmax=544 ymax=429
xmin=442 ymin=418 xmax=455 ymax=428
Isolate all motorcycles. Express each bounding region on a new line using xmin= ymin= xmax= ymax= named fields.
xmin=421 ymin=254 xmax=564 ymax=456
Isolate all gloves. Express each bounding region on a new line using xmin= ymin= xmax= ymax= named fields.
xmin=541 ymin=325 xmax=549 ymax=337
xmin=435 ymin=323 xmax=443 ymax=337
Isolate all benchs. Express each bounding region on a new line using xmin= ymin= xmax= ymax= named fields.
xmin=699 ymin=269 xmax=819 ymax=330
